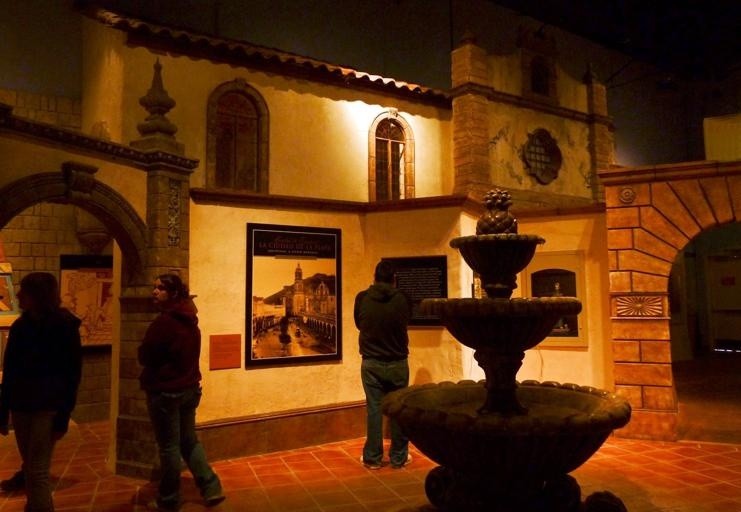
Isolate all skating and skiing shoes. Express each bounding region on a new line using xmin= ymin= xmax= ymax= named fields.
xmin=203 ymin=487 xmax=225 ymax=503
xmin=147 ymin=500 xmax=178 ymax=511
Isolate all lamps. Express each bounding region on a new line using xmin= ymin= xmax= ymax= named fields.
xmin=583 ymin=65 xmax=602 ymax=83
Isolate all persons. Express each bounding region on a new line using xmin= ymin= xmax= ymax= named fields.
xmin=136 ymin=274 xmax=226 ymax=511
xmin=353 ymin=260 xmax=412 ymax=472
xmin=0 ymin=271 xmax=83 ymax=512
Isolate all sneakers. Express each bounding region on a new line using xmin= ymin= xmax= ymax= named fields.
xmin=0 ymin=470 xmax=25 ymax=492
xmin=360 ymin=454 xmax=412 ymax=470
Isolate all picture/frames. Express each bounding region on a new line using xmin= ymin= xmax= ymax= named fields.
xmin=244 ymin=223 xmax=342 ymax=366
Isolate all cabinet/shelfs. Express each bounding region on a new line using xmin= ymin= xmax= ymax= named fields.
xmin=520 ymin=249 xmax=588 ymax=348
